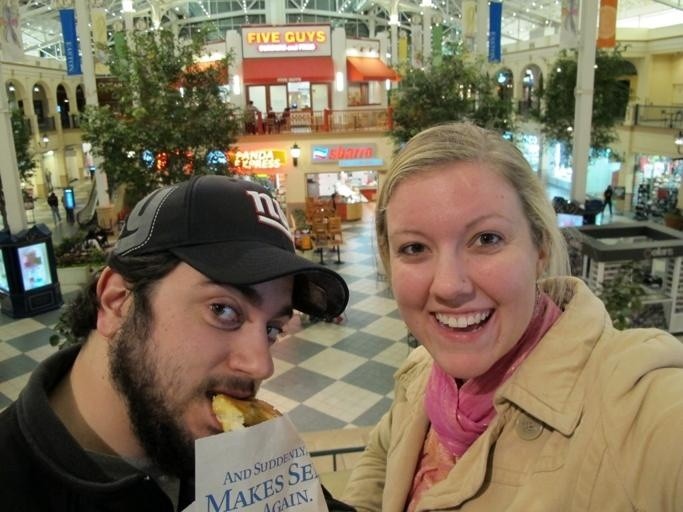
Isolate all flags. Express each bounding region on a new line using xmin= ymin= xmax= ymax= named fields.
xmin=0 ymin=0 xmax=26 ymax=61
xmin=58 ymin=9 xmax=83 ymax=77
xmin=90 ymin=8 xmax=109 ymax=65
xmin=383 ymin=0 xmax=503 ymax=81
xmin=556 ymin=0 xmax=579 ymax=51
xmin=596 ymin=0 xmax=618 ymax=50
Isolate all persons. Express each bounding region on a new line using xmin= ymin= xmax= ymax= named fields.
xmin=244 ymin=100 xmax=297 ymax=135
xmin=47 ymin=193 xmax=61 ymax=224
xmin=325 ymin=119 xmax=681 ymax=510
xmin=600 ymin=185 xmax=612 ymax=215
xmin=0 ymin=175 xmax=357 ymax=512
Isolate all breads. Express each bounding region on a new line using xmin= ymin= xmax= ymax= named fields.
xmin=212 ymin=394 xmax=283 ymax=432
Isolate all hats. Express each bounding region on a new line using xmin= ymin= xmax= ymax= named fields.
xmin=106 ymin=174 xmax=350 ymax=320
